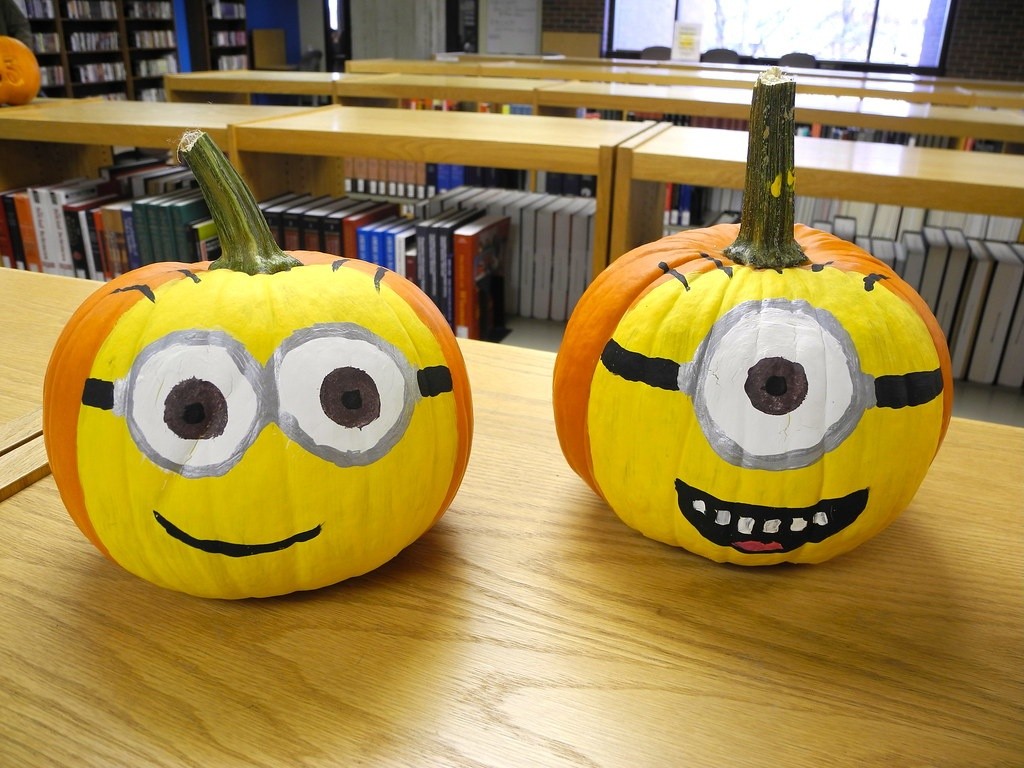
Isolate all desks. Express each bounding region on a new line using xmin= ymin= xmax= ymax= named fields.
xmin=1 ymin=266 xmax=109 ymax=502
xmin=0 ymin=338 xmax=1024 ymax=767
xmin=255 ymin=64 xmax=297 ymax=72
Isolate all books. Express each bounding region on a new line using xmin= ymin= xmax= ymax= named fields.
xmin=25 ymin=0 xmax=252 ymax=102
xmin=626 ymin=111 xmax=1024 ymax=388
xmin=0 ymin=98 xmax=623 ymax=342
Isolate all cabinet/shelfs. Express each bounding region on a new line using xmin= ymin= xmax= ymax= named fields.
xmin=1 ymin=51 xmax=1023 ymax=429
xmin=12 ymin=0 xmax=255 ymax=104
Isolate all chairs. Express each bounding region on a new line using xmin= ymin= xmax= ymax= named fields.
xmin=296 ymin=50 xmax=322 ymax=107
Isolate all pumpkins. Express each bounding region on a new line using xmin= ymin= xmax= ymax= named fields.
xmin=0 ymin=36 xmax=40 ymax=106
xmin=553 ymin=67 xmax=953 ymax=568
xmin=43 ymin=127 xmax=475 ymax=602
